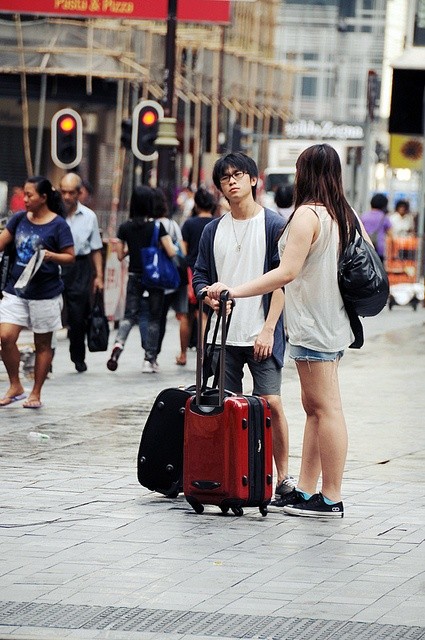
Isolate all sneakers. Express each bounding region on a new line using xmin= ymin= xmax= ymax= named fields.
xmin=284 ymin=494 xmax=345 ymax=520
xmin=267 ymin=486 xmax=309 ymax=515
xmin=106 ymin=343 xmax=123 ymax=371
xmin=275 ymin=478 xmax=298 ymax=500
xmin=143 ymin=360 xmax=159 ymax=372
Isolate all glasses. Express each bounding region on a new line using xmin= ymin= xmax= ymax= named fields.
xmin=218 ymin=168 xmax=248 ymax=182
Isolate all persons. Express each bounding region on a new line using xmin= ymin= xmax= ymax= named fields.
xmin=192 ymin=152 xmax=298 ymax=500
xmin=180 ymin=188 xmax=218 ymax=364
xmin=260 ymin=174 xmax=288 ymax=212
xmin=206 ymin=144 xmax=389 ymax=519
xmin=360 ymin=194 xmax=392 ymax=263
xmin=389 ymin=200 xmax=415 ymax=239
xmin=0 ymin=176 xmax=74 ymax=409
xmin=49 ymin=173 xmax=103 ymax=373
xmin=274 ymin=187 xmax=295 ymax=221
xmin=105 ymin=186 xmax=187 ymax=373
xmin=78 ymin=180 xmax=94 ymax=208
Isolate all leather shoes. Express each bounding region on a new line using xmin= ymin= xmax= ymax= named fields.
xmin=73 ymin=359 xmax=87 ymax=373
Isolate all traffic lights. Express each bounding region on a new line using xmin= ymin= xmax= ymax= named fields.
xmin=50 ymin=107 xmax=84 ymax=170
xmin=132 ymin=101 xmax=164 ymax=162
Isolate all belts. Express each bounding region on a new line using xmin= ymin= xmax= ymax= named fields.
xmin=75 ymin=253 xmax=90 ymax=261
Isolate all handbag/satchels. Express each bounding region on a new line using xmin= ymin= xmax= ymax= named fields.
xmin=86 ymin=287 xmax=110 ymax=351
xmin=0 ymin=212 xmax=26 ymax=291
xmin=139 ymin=217 xmax=182 ymax=291
xmin=367 ymin=208 xmax=386 ymax=243
xmin=337 ymin=196 xmax=389 ymax=318
xmin=170 ymin=219 xmax=183 ymax=268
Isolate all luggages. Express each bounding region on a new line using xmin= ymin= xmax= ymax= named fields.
xmin=182 ymin=288 xmax=274 ymax=519
xmin=136 ymin=301 xmax=237 ymax=501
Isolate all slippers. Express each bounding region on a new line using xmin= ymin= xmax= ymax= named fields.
xmin=22 ymin=400 xmax=42 ymax=409
xmin=176 ymin=355 xmax=185 ymax=366
xmin=0 ymin=395 xmax=27 ymax=404
